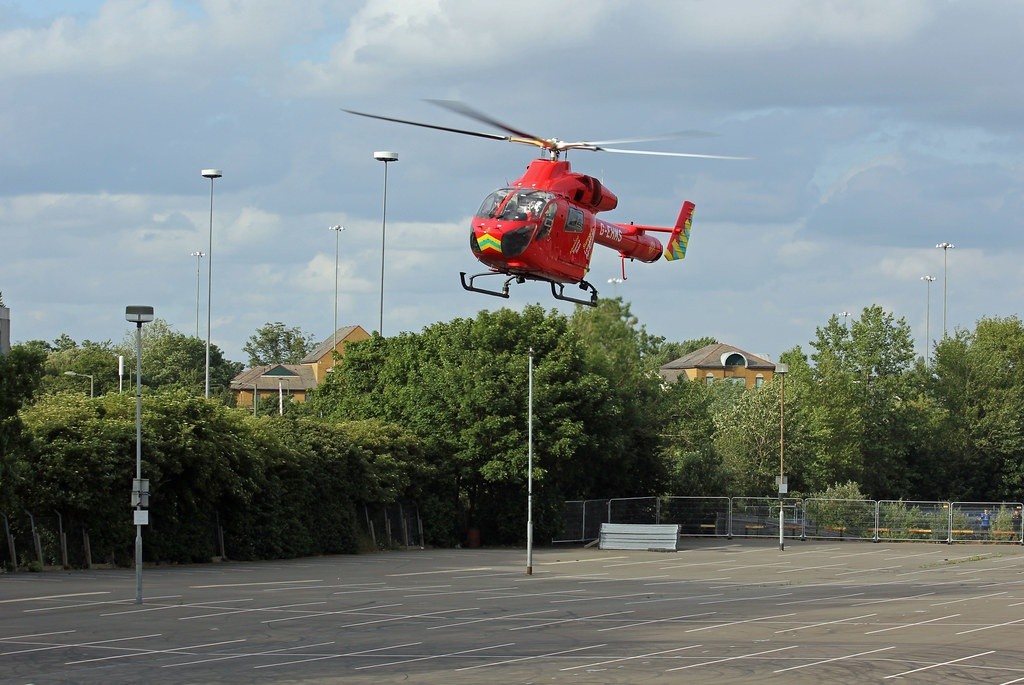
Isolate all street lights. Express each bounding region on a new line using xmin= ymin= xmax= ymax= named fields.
xmin=935 ymin=243 xmax=955 ymax=341
xmin=328 ymin=225 xmax=345 ymax=368
xmin=838 ymin=312 xmax=851 ymax=324
xmin=64 ymin=371 xmax=93 ymax=400
xmin=374 ymin=151 xmax=398 ymax=337
xmin=191 ymin=252 xmax=206 ymax=340
xmin=773 ymin=363 xmax=789 ymax=486
xmin=201 ymin=168 xmax=222 ymax=400
xmin=920 ymin=275 xmax=937 ymax=373
xmin=230 ymin=381 xmax=258 ymax=419
xmin=125 ymin=305 xmax=154 ymax=606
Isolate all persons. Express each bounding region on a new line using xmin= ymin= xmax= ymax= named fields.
xmin=979 ymin=508 xmax=992 ymax=540
xmin=526 ymin=198 xmax=548 ymax=220
xmin=1007 ymin=509 xmax=1022 ymax=544
xmin=488 ymin=196 xmax=507 ymax=217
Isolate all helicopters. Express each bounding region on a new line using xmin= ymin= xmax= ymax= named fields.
xmin=338 ymin=98 xmax=754 ymax=307
